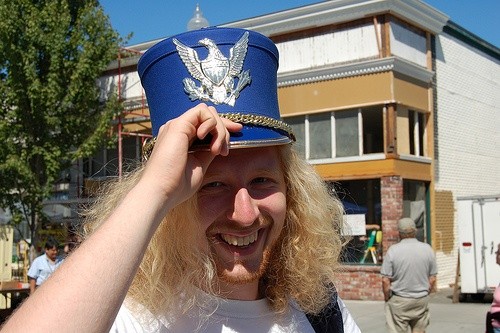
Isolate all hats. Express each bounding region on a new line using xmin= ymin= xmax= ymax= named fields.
xmin=137 ymin=25 xmax=297 ymax=156
xmin=397 ymin=218 xmax=418 ymax=233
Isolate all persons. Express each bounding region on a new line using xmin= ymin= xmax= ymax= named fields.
xmin=346 ymin=223 xmax=380 ymax=264
xmin=486 ymin=242 xmax=500 ymax=333
xmin=26 ymin=224 xmax=82 ymax=297
xmin=379 ymin=218 xmax=436 ymax=333
xmin=0 ymin=28 xmax=361 ymax=333
